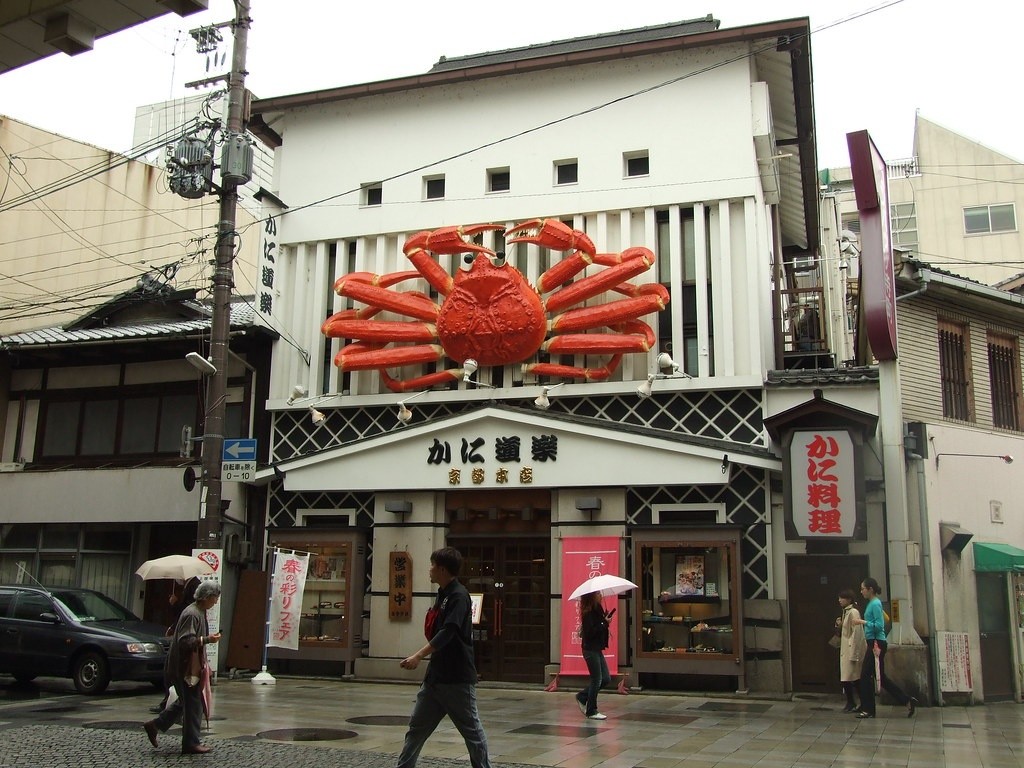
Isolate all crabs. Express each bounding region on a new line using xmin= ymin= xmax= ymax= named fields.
xmin=321 ymin=215 xmax=671 ymax=394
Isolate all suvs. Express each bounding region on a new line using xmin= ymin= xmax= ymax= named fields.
xmin=0 ymin=584 xmax=174 ymax=696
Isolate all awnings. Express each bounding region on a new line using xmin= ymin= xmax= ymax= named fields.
xmin=973 ymin=541 xmax=1024 ymax=572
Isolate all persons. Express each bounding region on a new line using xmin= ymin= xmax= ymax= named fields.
xmin=834 ymin=589 xmax=869 ymax=712
xmin=144 ymin=583 xmax=226 ymax=754
xmin=150 ymin=572 xmax=204 ymax=713
xmin=566 ymin=591 xmax=640 ymax=720
xmin=852 ymin=571 xmax=919 ymax=719
xmin=399 ymin=547 xmax=493 ymax=767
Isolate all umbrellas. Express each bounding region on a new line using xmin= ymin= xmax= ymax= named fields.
xmin=135 ymin=555 xmax=222 ymax=595
xmin=872 ymin=623 xmax=881 ymax=696
xmin=200 ymin=636 xmax=213 ymax=730
xmin=567 ymin=574 xmax=639 ymax=614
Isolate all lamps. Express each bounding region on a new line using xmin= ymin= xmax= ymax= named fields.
xmin=635 ymin=374 xmax=675 ymax=399
xmin=643 ymin=627 xmax=652 ymax=635
xmin=287 ymin=385 xmax=324 ymax=406
xmin=462 ymin=358 xmax=495 ymax=389
xmin=397 ymin=388 xmax=431 ymax=422
xmin=534 ymin=382 xmax=565 ymax=410
xmin=935 ymin=454 xmax=1013 ymax=472
xmin=385 ymin=500 xmax=413 ymax=513
xmin=655 ymin=353 xmax=693 ymax=379
xmin=574 ymin=497 xmax=601 ymax=521
xmin=307 ymin=393 xmax=342 ymax=428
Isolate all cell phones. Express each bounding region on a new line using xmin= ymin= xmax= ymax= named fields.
xmin=608 ymin=608 xmax=616 ymax=618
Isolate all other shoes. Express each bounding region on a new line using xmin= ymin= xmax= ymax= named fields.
xmin=586 ymin=712 xmax=607 ymax=719
xmin=576 ymin=694 xmax=587 ymax=716
xmin=182 ymin=745 xmax=212 ymax=753
xmin=148 ymin=705 xmax=162 ymax=712
xmin=143 ymin=721 xmax=159 ymax=747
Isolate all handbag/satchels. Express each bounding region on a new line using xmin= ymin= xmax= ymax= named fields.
xmin=882 ymin=610 xmax=889 ymax=624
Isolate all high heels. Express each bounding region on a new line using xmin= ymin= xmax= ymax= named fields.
xmin=907 ymin=697 xmax=919 ymax=718
xmin=856 ymin=711 xmax=875 ymax=718
